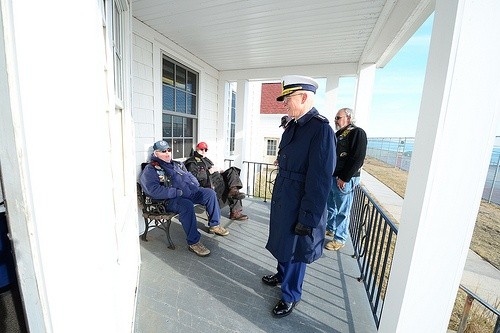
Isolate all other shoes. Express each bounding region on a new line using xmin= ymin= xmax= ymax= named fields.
xmin=188 ymin=241 xmax=211 ymax=257
xmin=229 ymin=210 xmax=248 ymax=220
xmin=325 ymin=240 xmax=345 ymax=251
xmin=227 ymin=187 xmax=246 ymax=201
xmin=208 ymin=225 xmax=229 ymax=236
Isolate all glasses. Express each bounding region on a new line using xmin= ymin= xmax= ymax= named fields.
xmin=200 ymin=149 xmax=208 ymax=152
xmin=283 ymin=92 xmax=303 ymax=104
xmin=156 ymin=149 xmax=171 ymax=153
xmin=335 ymin=115 xmax=347 ymax=120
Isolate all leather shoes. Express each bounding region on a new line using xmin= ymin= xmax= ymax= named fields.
xmin=272 ymin=299 xmax=301 ymax=317
xmin=262 ymin=272 xmax=283 ymax=287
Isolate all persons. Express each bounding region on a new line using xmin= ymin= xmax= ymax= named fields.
xmin=324 ymin=108 xmax=368 ymax=251
xmin=138 ymin=140 xmax=229 ymax=257
xmin=261 ymin=75 xmax=336 ymax=318
xmin=182 ymin=142 xmax=248 ymax=221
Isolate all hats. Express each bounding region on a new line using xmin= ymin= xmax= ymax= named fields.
xmin=196 ymin=142 xmax=208 ymax=149
xmin=276 ymin=75 xmax=319 ymax=102
xmin=153 ymin=140 xmax=171 ymax=150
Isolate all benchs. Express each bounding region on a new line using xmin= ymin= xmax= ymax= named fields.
xmin=137 ymin=162 xmax=242 ymax=250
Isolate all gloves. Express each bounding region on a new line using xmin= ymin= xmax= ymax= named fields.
xmin=295 ymin=222 xmax=312 ymax=236
